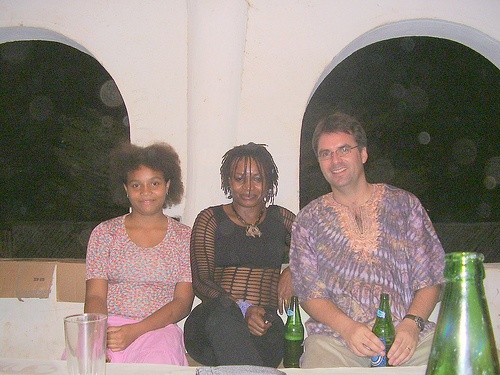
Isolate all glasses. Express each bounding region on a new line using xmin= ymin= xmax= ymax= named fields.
xmin=316 ymin=145 xmax=357 ymax=161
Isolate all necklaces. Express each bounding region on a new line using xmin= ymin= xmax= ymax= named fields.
xmin=233 ymin=206 xmax=268 ymax=238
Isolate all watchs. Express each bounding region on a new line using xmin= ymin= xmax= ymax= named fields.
xmin=403 ymin=314 xmax=425 ymax=331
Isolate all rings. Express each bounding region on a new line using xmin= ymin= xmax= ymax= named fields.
xmin=406 ymin=347 xmax=410 ymax=351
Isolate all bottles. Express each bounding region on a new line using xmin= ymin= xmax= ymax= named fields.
xmin=370 ymin=293 xmax=396 ymax=367
xmin=282 ymin=295 xmax=304 ymax=368
xmin=425 ymin=251 xmax=500 ymax=375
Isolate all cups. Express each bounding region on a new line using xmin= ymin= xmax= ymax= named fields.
xmin=63 ymin=313 xmax=108 ymax=375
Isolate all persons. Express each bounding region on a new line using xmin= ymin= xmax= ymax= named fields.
xmin=61 ymin=141 xmax=195 ymax=366
xmin=289 ymin=114 xmax=446 ymax=369
xmin=184 ymin=141 xmax=298 ymax=366
